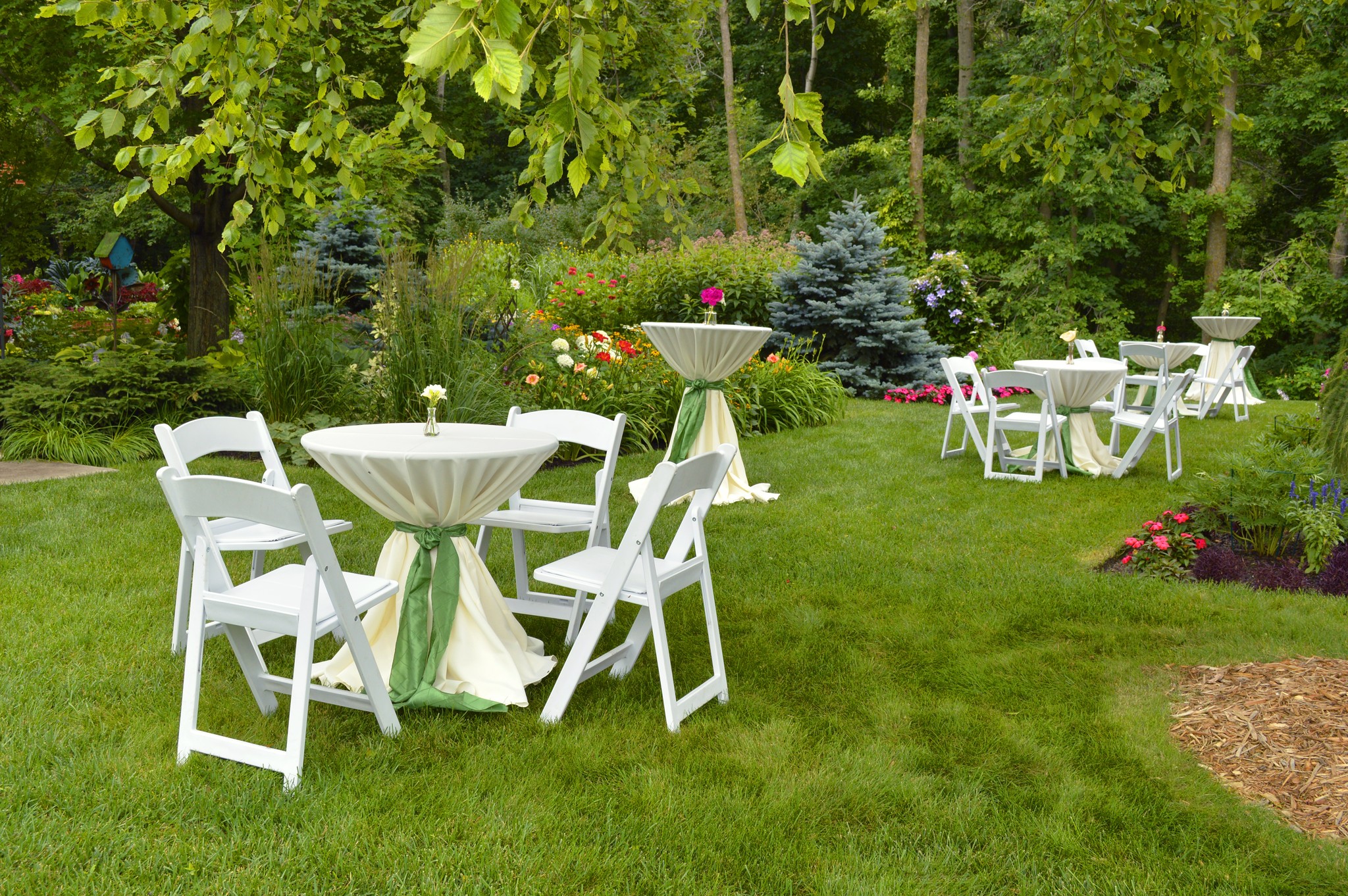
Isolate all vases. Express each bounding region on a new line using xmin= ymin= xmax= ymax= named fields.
xmin=704 ymin=302 xmax=717 ymax=325
xmin=1157 ymin=330 xmax=1164 ymax=343
xmin=423 ymin=407 xmax=439 ymax=436
xmin=1066 ymin=342 xmax=1074 ymax=365
xmin=1221 ymin=309 xmax=1229 ymax=316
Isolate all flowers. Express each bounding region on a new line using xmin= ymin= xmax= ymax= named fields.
xmin=1156 ymin=321 xmax=1166 ymax=332
xmin=1222 ymin=302 xmax=1230 ymax=310
xmin=422 ymin=380 xmax=448 ymax=408
xmin=1059 ymin=327 xmax=1078 ymax=342
xmin=701 ymin=285 xmax=725 ymax=309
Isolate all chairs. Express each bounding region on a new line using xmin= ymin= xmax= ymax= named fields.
xmin=1074 ymin=338 xmax=1114 ymax=402
xmin=156 ymin=466 xmax=403 ymax=788
xmin=1118 ymin=341 xmax=1172 ymax=418
xmin=1112 ymin=368 xmax=1195 ymax=483
xmin=476 ymin=405 xmax=630 ymax=648
xmin=543 ymin=441 xmax=739 ymax=737
xmin=1167 ymin=340 xmax=1211 ymax=411
xmin=980 ymin=368 xmax=1069 ymax=484
xmin=154 ymin=412 xmax=353 ymax=659
xmin=941 ymin=355 xmax=1019 ymax=462
xmin=1194 ymin=345 xmax=1256 ymax=423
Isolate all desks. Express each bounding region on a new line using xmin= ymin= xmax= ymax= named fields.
xmin=1184 ymin=316 xmax=1266 ymax=405
xmin=1119 ymin=341 xmax=1201 ymax=416
xmin=301 ymin=422 xmax=556 ymax=709
xmin=1009 ymin=357 xmax=1130 ymax=477
xmin=629 ymin=321 xmax=781 ymax=507
